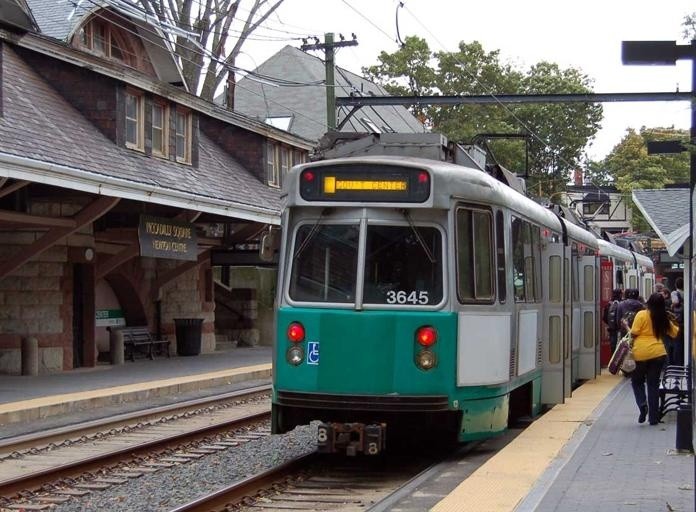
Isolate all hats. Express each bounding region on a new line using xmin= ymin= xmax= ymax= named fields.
xmin=612 ymin=287 xmax=640 ymax=299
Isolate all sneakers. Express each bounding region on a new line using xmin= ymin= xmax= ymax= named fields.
xmin=637 ymin=406 xmax=659 ymax=426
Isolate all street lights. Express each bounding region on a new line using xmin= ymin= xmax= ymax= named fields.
xmin=619 ymin=34 xmax=695 ymax=454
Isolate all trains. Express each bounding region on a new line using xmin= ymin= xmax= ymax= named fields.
xmin=267 ymin=155 xmax=658 ymax=461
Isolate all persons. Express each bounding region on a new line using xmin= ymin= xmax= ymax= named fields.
xmin=601 ymin=276 xmax=687 ymax=425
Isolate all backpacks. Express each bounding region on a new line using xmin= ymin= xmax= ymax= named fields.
xmin=672 ymin=290 xmax=684 ymax=324
xmin=608 ymin=300 xmax=641 ymax=332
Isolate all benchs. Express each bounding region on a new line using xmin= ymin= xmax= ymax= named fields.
xmin=105 ymin=325 xmax=171 ymax=363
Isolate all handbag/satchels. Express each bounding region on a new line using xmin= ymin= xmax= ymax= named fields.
xmin=607 ymin=330 xmax=637 ymax=376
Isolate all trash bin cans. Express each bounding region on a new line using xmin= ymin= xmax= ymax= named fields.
xmin=172 ymin=317 xmax=205 ymax=357
